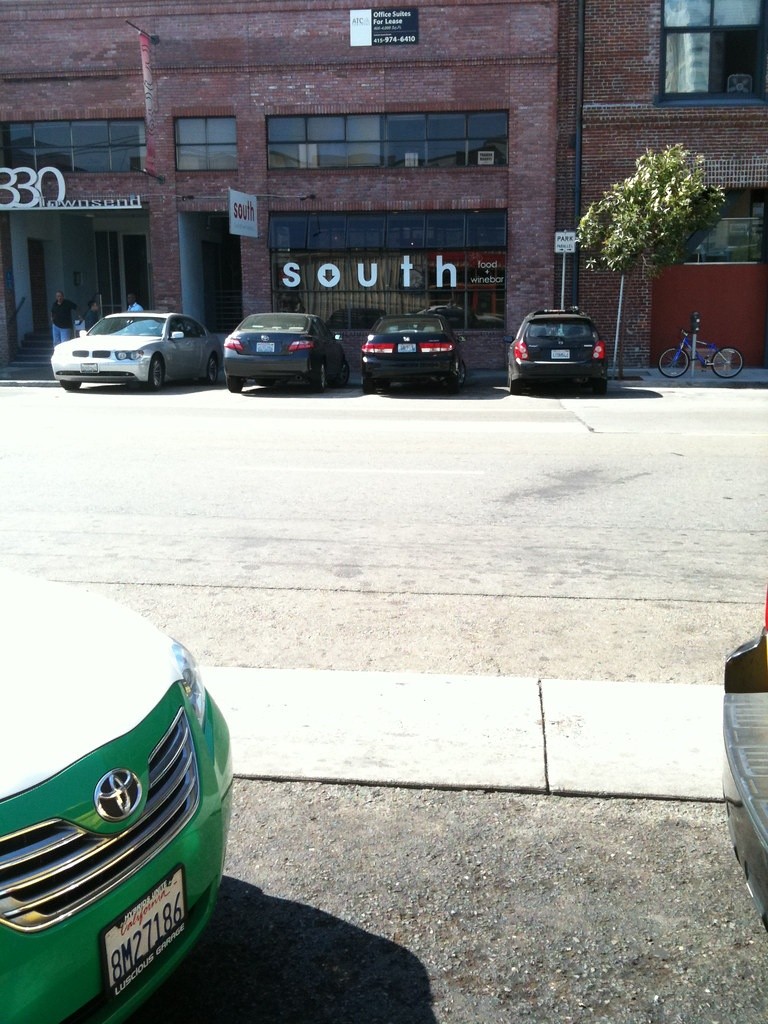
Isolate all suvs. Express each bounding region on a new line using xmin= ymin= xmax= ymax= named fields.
xmin=502 ymin=306 xmax=607 ymax=394
xmin=721 ymin=581 xmax=768 ymax=930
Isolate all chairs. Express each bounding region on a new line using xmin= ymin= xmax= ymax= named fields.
xmin=422 ymin=325 xmax=435 ymax=331
xmin=562 ymin=325 xmax=583 ymax=336
xmin=387 ymin=324 xmax=398 ymax=332
xmin=531 ymin=327 xmax=546 ymax=336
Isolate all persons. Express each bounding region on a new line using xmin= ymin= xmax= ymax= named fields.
xmin=51 ymin=291 xmax=82 ymax=348
xmin=126 ymin=292 xmax=144 ymax=312
xmin=84 ymin=299 xmax=99 ymax=332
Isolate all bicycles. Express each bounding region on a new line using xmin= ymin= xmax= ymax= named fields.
xmin=657 ymin=325 xmax=744 ymax=378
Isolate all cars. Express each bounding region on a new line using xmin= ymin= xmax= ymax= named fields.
xmin=324 ymin=307 xmax=386 ymax=330
xmin=0 ymin=564 xmax=232 ymax=1024
xmin=50 ymin=311 xmax=223 ymax=391
xmin=224 ymin=312 xmax=349 ymax=394
xmin=361 ymin=313 xmax=465 ymax=394
xmin=417 ymin=305 xmax=478 ymax=328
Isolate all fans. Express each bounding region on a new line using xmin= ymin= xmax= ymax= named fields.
xmin=727 ymin=73 xmax=753 ymax=94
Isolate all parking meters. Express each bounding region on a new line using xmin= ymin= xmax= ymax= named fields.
xmin=690 ymin=311 xmax=702 ymax=377
